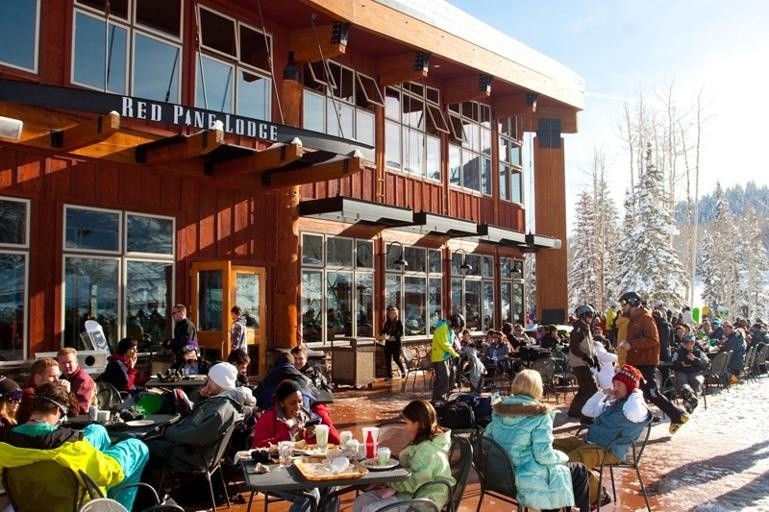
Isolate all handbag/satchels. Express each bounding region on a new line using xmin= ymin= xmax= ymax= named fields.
xmin=78 ymin=470 xmax=185 ymax=512
xmin=430 ymin=392 xmax=492 ymax=430
xmin=134 ymin=391 xmax=163 ymax=413
xmin=313 ymin=387 xmax=334 ymax=405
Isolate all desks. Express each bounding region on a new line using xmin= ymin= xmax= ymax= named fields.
xmin=140 ymin=374 xmax=208 ymax=400
xmin=240 ymin=449 xmax=412 ymax=512
xmin=59 ymin=413 xmax=181 ymax=444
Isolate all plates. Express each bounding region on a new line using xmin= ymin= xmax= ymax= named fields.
xmin=150 ymin=375 xmax=165 ymax=379
xmin=284 ymin=440 xmax=306 ymax=452
xmin=358 ymin=459 xmax=400 ymax=470
xmin=188 ymin=375 xmax=208 ymax=379
xmin=127 ymin=420 xmax=154 ymax=426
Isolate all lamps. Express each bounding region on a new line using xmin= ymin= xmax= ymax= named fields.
xmin=497 ymin=254 xmax=523 ymax=274
xmin=446 ymin=248 xmax=474 ymax=271
xmin=377 ymin=240 xmax=408 ymax=265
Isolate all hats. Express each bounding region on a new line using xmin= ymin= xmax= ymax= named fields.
xmin=182 ymin=340 xmax=198 ymax=352
xmin=612 ymin=365 xmax=641 ymax=394
xmin=0 ymin=375 xmax=23 ymax=400
xmin=208 ymin=362 xmax=238 ymax=391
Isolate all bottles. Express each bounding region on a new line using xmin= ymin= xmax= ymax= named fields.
xmin=366 ymin=431 xmax=376 ymax=459
xmin=89 ymin=397 xmax=98 ymax=421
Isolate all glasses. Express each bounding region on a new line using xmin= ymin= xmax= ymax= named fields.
xmin=585 ymin=312 xmax=593 ymax=318
xmin=4 ymin=390 xmax=23 ymax=401
xmin=183 ymin=345 xmax=195 ymax=352
xmin=620 ymin=301 xmax=627 ymax=306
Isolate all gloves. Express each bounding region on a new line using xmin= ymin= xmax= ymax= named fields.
xmin=592 ymin=355 xmax=601 ymax=372
xmin=583 ymin=354 xmax=594 ymax=367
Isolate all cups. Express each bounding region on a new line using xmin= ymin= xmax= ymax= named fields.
xmin=97 ymin=411 xmax=111 ymax=421
xmin=362 ymin=427 xmax=379 ymax=459
xmin=379 ymin=446 xmax=392 ymax=466
xmin=345 ymin=439 xmax=360 ymax=453
xmin=340 ymin=431 xmax=354 ymax=448
xmin=619 ymin=340 xmax=627 ymax=349
xmin=278 ymin=443 xmax=292 ymax=469
xmin=167 ymin=368 xmax=176 ymax=375
xmin=316 ymin=425 xmax=330 ymax=446
xmin=181 ymin=368 xmax=190 ymax=378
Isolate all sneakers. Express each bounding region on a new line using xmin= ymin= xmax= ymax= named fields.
xmin=669 ymin=412 xmax=689 ymax=434
xmin=681 ymin=387 xmax=698 ymax=414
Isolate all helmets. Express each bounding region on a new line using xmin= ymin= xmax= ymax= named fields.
xmin=618 ymin=292 xmax=642 ymax=317
xmin=576 ymin=305 xmax=594 ymax=326
xmin=682 ymin=334 xmax=696 ymax=343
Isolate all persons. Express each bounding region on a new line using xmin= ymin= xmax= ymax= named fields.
xmin=616 ymin=291 xmax=690 ymax=435
xmin=430 ymin=312 xmax=464 ymax=398
xmin=169 ymin=303 xmax=200 ymax=370
xmin=566 ymin=306 xmax=601 ymax=426
xmin=551 ymin=363 xmax=654 ymax=511
xmin=351 ymin=397 xmax=457 ymax=511
xmin=303 ymin=303 xmax=372 ymax=338
xmin=379 ymin=306 xmax=406 ymax=381
xmin=480 ymin=368 xmax=611 ymax=511
xmin=72 ymin=307 xmax=165 ymax=336
xmin=229 ymin=306 xmax=249 ymax=358
xmin=0 ymin=337 xmax=339 ymax=511
xmin=460 ymin=303 xmax=768 ymax=414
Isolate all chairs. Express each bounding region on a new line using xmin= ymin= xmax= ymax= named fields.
xmin=471 ymin=414 xmax=654 ymax=512
xmin=357 ymin=416 xmax=473 ymax=511
xmin=402 ymin=331 xmax=768 ymax=414
xmin=0 ymin=357 xmax=327 ymax=511
